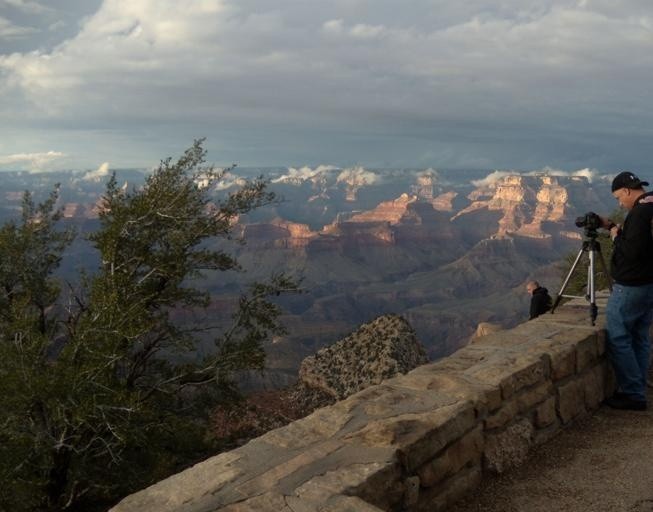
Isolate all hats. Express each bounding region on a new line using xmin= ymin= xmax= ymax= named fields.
xmin=612 ymin=171 xmax=648 ymax=192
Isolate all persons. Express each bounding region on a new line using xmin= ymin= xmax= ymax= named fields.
xmin=599 ymin=171 xmax=652 ymax=411
xmin=527 ymin=280 xmax=553 ymax=321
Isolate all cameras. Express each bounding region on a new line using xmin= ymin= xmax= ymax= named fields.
xmin=576 ymin=212 xmax=602 ymax=228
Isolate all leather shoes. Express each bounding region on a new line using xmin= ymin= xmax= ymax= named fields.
xmin=601 ymin=389 xmax=647 ymax=411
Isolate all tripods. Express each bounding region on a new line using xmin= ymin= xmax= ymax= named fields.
xmin=551 ymin=238 xmax=613 ymax=326
